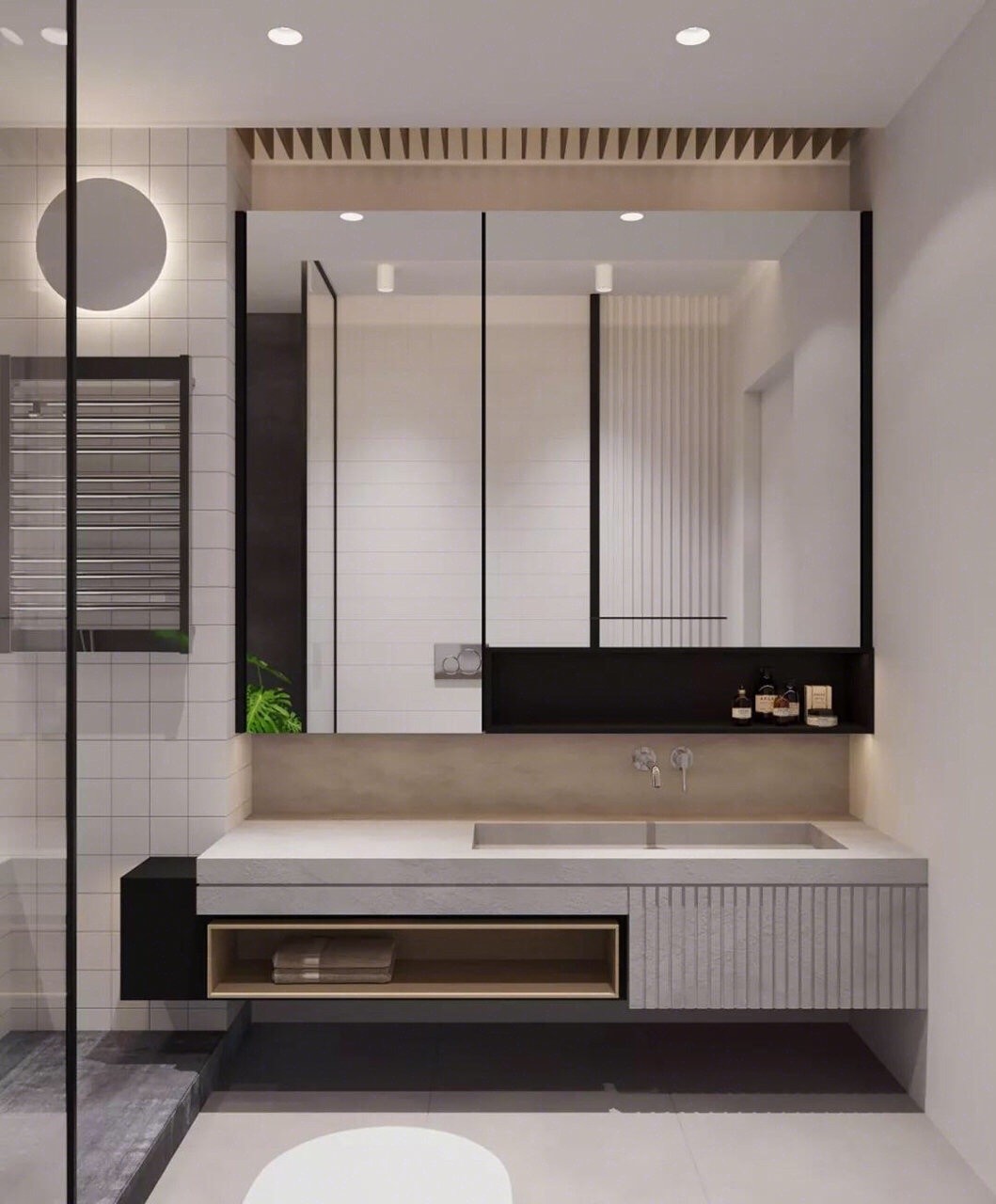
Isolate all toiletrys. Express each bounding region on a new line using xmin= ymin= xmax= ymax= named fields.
xmin=755 ymin=674 xmax=801 ymax=723
xmin=729 ymin=683 xmax=753 ymax=726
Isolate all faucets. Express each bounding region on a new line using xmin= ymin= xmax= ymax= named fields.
xmin=633 ymin=746 xmax=663 ymax=789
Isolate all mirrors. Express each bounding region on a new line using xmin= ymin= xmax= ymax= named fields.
xmin=246 ymin=208 xmax=481 ymax=733
xmin=485 ymin=208 xmax=861 ymax=649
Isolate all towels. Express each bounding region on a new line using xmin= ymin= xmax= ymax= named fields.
xmin=270 ymin=937 xmax=399 ymax=984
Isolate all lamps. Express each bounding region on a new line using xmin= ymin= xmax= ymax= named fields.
xmin=36 ymin=174 xmax=168 ymax=316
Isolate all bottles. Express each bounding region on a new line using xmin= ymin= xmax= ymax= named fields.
xmin=772 ymin=693 xmax=789 ymax=726
xmin=752 ymin=676 xmax=778 ymax=726
xmin=731 ymin=684 xmax=752 ymax=727
xmin=782 ymin=680 xmax=799 ymax=724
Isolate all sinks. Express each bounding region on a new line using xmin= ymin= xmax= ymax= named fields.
xmin=469 ymin=820 xmax=658 ymax=849
xmin=647 ymin=819 xmax=850 ymax=849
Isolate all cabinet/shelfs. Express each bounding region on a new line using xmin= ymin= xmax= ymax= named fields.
xmin=120 ymin=856 xmax=928 ymax=1009
